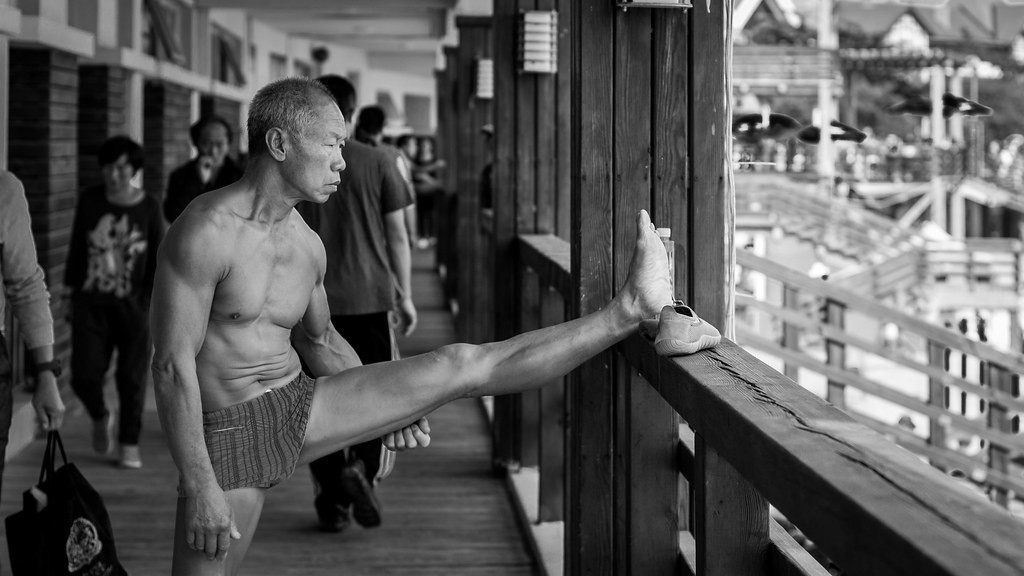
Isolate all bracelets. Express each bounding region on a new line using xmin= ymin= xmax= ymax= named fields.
xmin=63 ymin=295 xmax=71 ymax=299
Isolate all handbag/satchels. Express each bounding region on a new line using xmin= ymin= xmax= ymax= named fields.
xmin=5 ymin=429 xmax=128 ymax=576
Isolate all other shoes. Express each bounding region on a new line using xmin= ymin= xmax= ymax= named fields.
xmin=316 ymin=506 xmax=351 ymax=531
xmin=343 ymin=462 xmax=383 ymax=529
xmin=118 ymin=442 xmax=142 ymax=469
xmin=91 ymin=407 xmax=114 ymax=453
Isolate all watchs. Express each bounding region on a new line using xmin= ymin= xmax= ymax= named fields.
xmin=35 ymin=359 xmax=63 ymax=377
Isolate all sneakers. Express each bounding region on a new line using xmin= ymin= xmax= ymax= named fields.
xmin=654 ymin=299 xmax=721 ymax=355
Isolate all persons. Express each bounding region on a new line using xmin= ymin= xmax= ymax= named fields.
xmin=163 ymin=115 xmax=245 ymax=224
xmin=357 ymin=106 xmax=438 ymax=251
xmin=478 ymin=123 xmax=494 ymax=209
xmin=60 ymin=135 xmax=165 ymax=467
xmin=0 ymin=171 xmax=65 ymax=494
xmin=295 ymin=74 xmax=418 ymax=534
xmin=149 ymin=77 xmax=675 ymax=576
